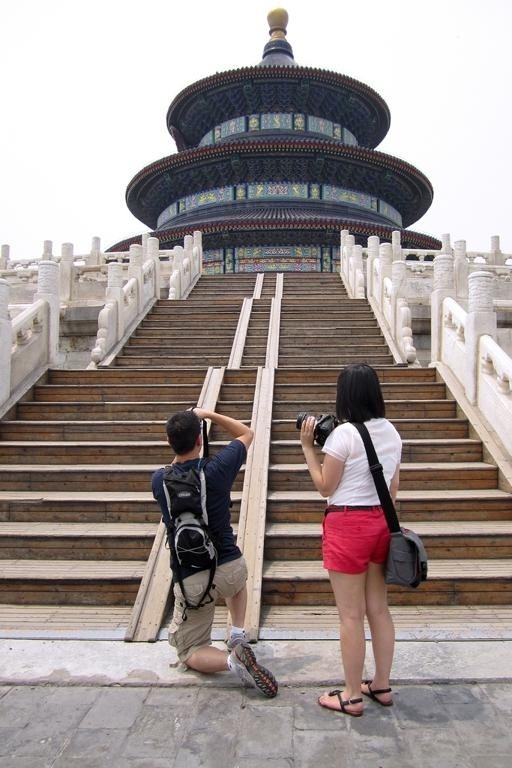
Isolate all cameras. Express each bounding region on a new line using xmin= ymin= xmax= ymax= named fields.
xmin=295 ymin=411 xmax=339 ymax=448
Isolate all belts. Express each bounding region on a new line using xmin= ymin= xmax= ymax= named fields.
xmin=324 ymin=505 xmax=379 ymax=517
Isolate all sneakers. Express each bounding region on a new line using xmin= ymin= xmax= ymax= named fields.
xmin=224 ymin=634 xmax=278 ymax=698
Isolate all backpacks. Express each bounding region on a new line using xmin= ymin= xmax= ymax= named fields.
xmin=160 ymin=458 xmax=218 ymax=569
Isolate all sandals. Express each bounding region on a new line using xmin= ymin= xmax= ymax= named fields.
xmin=318 ymin=690 xmax=364 ymax=717
xmin=361 ymin=680 xmax=394 ymax=705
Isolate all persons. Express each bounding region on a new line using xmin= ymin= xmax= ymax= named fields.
xmin=299 ymin=361 xmax=404 ymax=717
xmin=150 ymin=407 xmax=278 ymax=698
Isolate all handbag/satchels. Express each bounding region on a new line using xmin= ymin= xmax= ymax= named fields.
xmin=384 ymin=526 xmax=427 ymax=589
